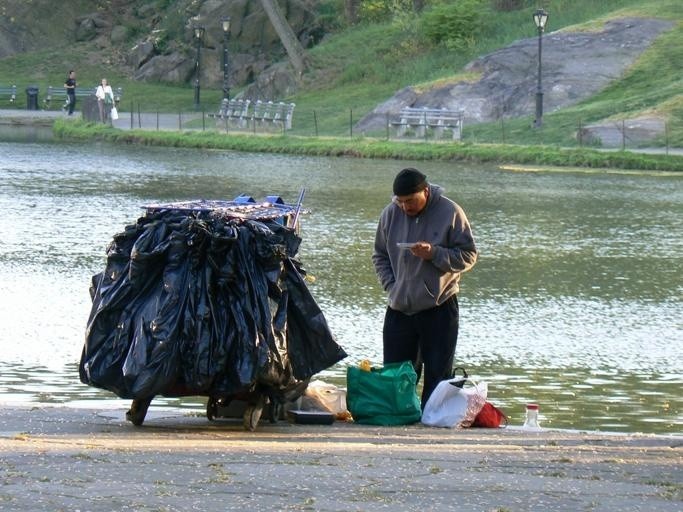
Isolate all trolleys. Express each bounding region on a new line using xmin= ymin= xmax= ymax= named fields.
xmin=127 ymin=193 xmax=303 ymax=432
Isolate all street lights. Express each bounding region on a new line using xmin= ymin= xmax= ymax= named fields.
xmin=191 ymin=22 xmax=205 ymax=110
xmin=220 ymin=15 xmax=232 ymax=98
xmin=532 ymin=8 xmax=549 ymax=128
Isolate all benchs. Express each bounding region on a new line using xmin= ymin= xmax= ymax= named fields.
xmin=208 ymin=98 xmax=296 ymax=132
xmin=391 ymin=106 xmax=465 ymax=140
xmin=0 ymin=85 xmax=17 ymax=104
xmin=42 ymin=86 xmax=122 ymax=112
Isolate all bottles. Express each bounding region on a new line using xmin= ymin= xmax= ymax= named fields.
xmin=522 ymin=404 xmax=541 ymax=449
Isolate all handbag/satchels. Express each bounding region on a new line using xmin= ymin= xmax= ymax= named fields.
xmin=346 ymin=360 xmax=422 ymax=426
xmin=110 ymin=107 xmax=119 ymax=120
xmin=473 ymin=402 xmax=508 ymax=430
xmin=105 ymin=94 xmax=112 ymax=105
xmin=424 ymin=371 xmax=486 ymax=428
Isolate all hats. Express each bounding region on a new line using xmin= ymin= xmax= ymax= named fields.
xmin=393 ymin=167 xmax=429 ymax=195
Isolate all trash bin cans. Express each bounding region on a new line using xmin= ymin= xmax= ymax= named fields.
xmin=26 ymin=87 xmax=39 ymax=110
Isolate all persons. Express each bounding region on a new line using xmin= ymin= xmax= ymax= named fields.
xmin=94 ymin=78 xmax=116 ymax=124
xmin=60 ymin=71 xmax=81 ymax=116
xmin=371 ymin=166 xmax=477 ymax=417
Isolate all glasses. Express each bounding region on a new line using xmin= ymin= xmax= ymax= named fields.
xmin=394 ymin=191 xmax=424 ymax=206
